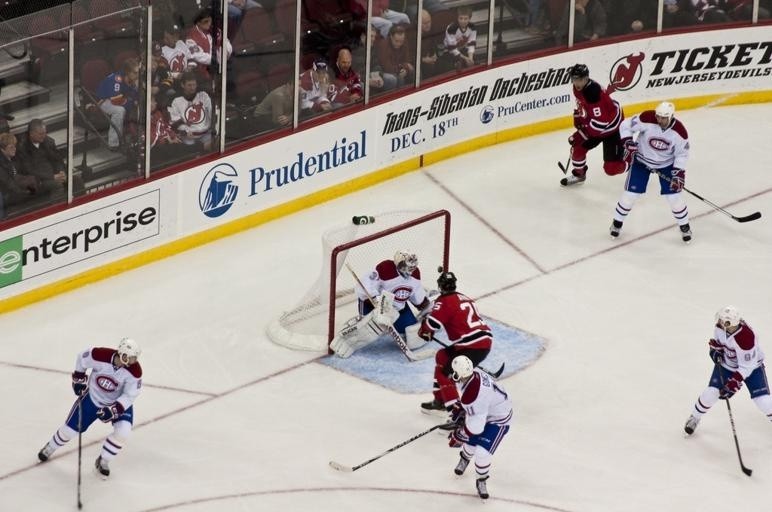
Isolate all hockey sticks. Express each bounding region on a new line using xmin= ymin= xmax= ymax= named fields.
xmin=329 ymin=422 xmax=454 ymax=473
xmin=636 ymin=155 xmax=761 ymax=223
xmin=77 ymin=395 xmax=82 ymax=508
xmin=432 ymin=335 xmax=505 ymax=378
xmin=716 ymin=364 xmax=752 ymax=477
xmin=557 ymin=147 xmax=575 ymax=173
xmin=344 ymin=261 xmax=434 ymax=362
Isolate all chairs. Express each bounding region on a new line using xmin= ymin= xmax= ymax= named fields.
xmin=228 ymin=1 xmax=356 ymax=106
xmin=428 ymin=1 xmax=457 ymax=43
xmin=16 ymin=0 xmax=142 ymax=92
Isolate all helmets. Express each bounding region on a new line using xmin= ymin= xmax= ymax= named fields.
xmin=657 ymin=102 xmax=675 ymax=124
xmin=394 ymin=249 xmax=417 ymax=271
xmin=436 ymin=271 xmax=457 ymax=290
xmin=569 ymin=63 xmax=590 ymax=80
xmin=715 ymin=304 xmax=741 ymax=327
xmin=118 ymin=336 xmax=141 ymax=364
xmin=450 ymin=355 xmax=474 ymax=381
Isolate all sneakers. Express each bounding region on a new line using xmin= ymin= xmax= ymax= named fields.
xmin=454 ymin=457 xmax=470 ymax=475
xmin=560 ymin=170 xmax=586 ymax=186
xmin=679 ymin=222 xmax=692 ymax=241
xmin=94 ymin=455 xmax=111 ymax=476
xmin=476 ymin=479 xmax=489 ymax=498
xmin=611 ymin=216 xmax=623 ymax=237
xmin=421 ymin=399 xmax=448 ymax=411
xmin=38 ymin=441 xmax=55 ymax=462
xmin=684 ymin=413 xmax=701 ymax=435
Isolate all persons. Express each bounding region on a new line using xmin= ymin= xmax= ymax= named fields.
xmin=37 ymin=335 xmax=143 ymax=476
xmin=682 ymin=307 xmax=771 ymax=437
xmin=607 ymin=100 xmax=696 ymax=241
xmin=445 ymin=355 xmax=514 ymax=500
xmin=418 ymin=271 xmax=492 ymax=413
xmin=354 ymin=247 xmax=439 ymax=337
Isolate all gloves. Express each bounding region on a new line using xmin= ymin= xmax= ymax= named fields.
xmin=95 ymin=402 xmax=123 ymax=423
xmin=708 ymin=338 xmax=725 ymax=363
xmin=447 ymin=427 xmax=469 ymax=449
xmin=718 ymin=374 xmax=742 ymax=400
xmin=573 ymin=108 xmax=586 ymax=128
xmin=568 ymin=128 xmax=589 ymax=145
xmin=449 ymin=401 xmax=465 ymax=421
xmin=620 ymin=140 xmax=639 ymax=164
xmin=71 ymin=372 xmax=88 ymax=395
xmin=670 ymin=166 xmax=685 ymax=193
